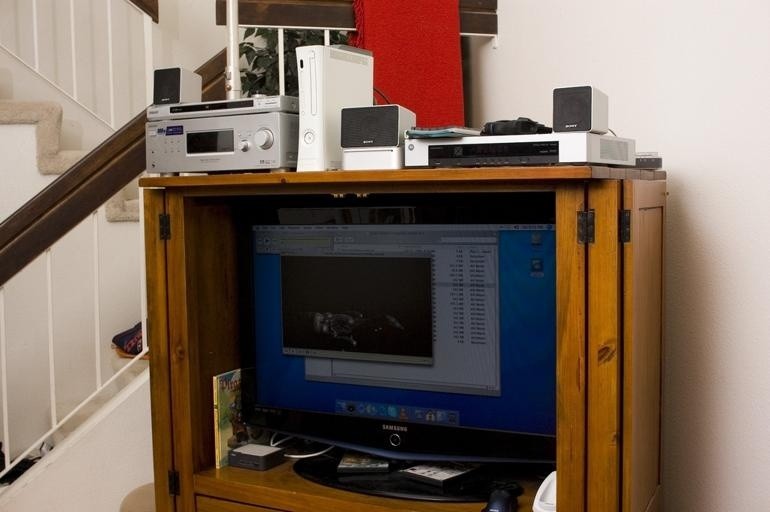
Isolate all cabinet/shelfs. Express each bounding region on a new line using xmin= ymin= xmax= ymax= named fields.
xmin=137 ymin=167 xmax=667 ymax=512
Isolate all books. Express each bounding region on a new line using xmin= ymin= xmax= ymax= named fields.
xmin=212 ymin=365 xmax=265 ymax=470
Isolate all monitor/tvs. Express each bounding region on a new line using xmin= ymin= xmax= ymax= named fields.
xmin=234 ymin=205 xmax=556 ymax=503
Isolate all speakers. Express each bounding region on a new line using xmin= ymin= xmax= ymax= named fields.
xmin=554 ymin=86 xmax=609 ymax=134
xmin=154 ymin=67 xmax=202 ymax=104
xmin=340 ymin=105 xmax=416 ymax=170
